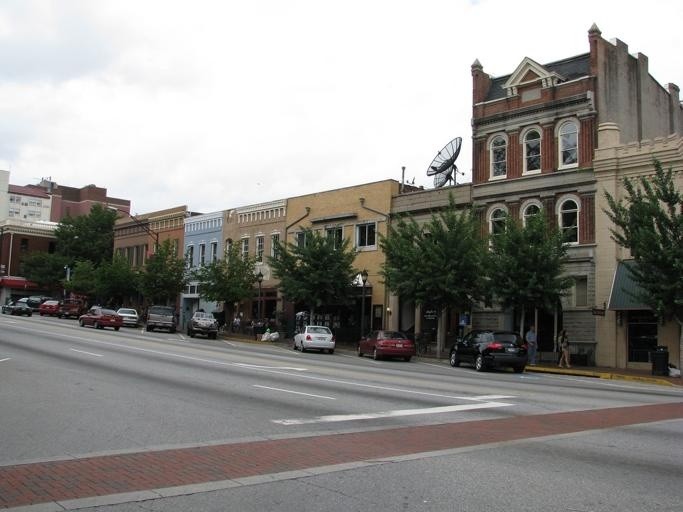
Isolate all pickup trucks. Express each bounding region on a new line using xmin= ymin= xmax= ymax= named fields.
xmin=144 ymin=306 xmax=178 ymax=333
xmin=186 ymin=311 xmax=218 ymax=339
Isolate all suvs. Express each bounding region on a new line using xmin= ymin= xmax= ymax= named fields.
xmin=26 ymin=296 xmax=54 ymax=312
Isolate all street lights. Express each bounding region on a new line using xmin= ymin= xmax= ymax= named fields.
xmin=255 ymin=269 xmax=263 ymax=325
xmin=106 ymin=206 xmax=158 ymax=306
xmin=73 ymin=234 xmax=105 ymax=305
xmin=359 ymin=268 xmax=368 ymax=340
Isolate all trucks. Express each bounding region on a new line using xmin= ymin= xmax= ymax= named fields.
xmin=57 ymin=292 xmax=96 ymax=318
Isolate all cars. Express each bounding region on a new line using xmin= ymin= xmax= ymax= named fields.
xmin=78 ymin=307 xmax=122 ymax=331
xmin=114 ymin=308 xmax=141 ymax=328
xmin=291 ymin=326 xmax=335 ymax=354
xmin=0 ymin=300 xmax=32 ymax=317
xmin=357 ymin=329 xmax=414 ymax=362
xmin=17 ymin=297 xmax=28 ymax=303
xmin=241 ymin=319 xmax=271 ymax=335
xmin=295 ymin=311 xmax=323 ymax=328
xmin=233 ymin=318 xmax=252 ymax=333
xmin=4 ymin=293 xmax=31 ymax=305
xmin=38 ymin=300 xmax=61 ymax=317
xmin=448 ymin=329 xmax=528 ymax=374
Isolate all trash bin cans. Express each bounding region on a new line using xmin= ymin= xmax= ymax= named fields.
xmin=651 ymin=346 xmax=669 ymax=376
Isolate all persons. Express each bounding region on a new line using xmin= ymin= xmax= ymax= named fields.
xmin=525 ymin=326 xmax=538 ymax=366
xmin=559 ymin=328 xmax=573 ymax=369
xmin=233 ymin=312 xmax=242 ymax=333
